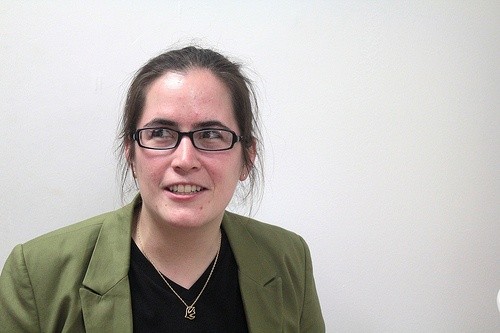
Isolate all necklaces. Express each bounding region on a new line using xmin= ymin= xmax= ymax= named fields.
xmin=137 ymin=206 xmax=222 ymax=320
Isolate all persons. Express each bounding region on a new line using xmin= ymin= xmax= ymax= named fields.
xmin=0 ymin=43 xmax=328 ymax=333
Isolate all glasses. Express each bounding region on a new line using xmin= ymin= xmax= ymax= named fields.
xmin=131 ymin=126 xmax=245 ymax=150
